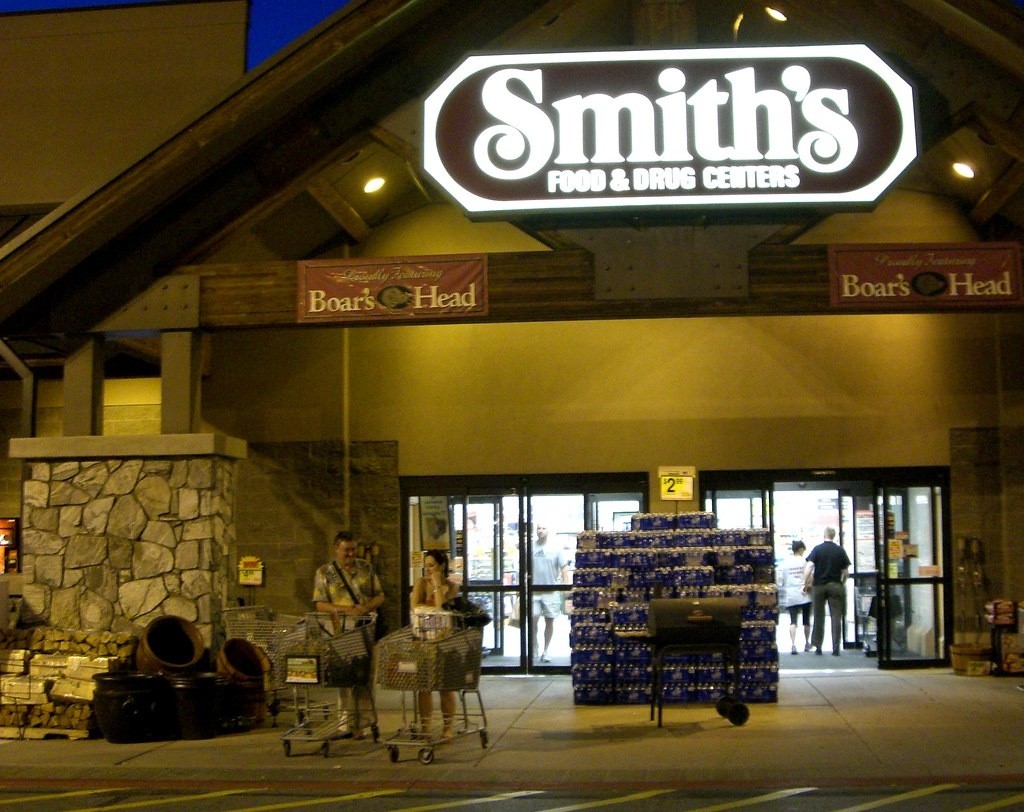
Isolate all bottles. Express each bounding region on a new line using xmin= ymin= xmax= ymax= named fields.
xmin=567 ymin=512 xmax=779 ymax=702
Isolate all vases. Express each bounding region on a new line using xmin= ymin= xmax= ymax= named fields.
xmin=92 ymin=615 xmax=270 ymax=743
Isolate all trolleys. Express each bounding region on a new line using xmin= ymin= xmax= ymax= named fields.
xmin=373 ymin=610 xmax=489 ymax=765
xmin=222 ymin=604 xmax=304 ymax=728
xmin=270 ymin=611 xmax=377 ymax=757
xmin=853 ymin=585 xmax=907 ymax=659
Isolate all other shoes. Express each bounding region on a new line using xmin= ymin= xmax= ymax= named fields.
xmin=419 ymin=727 xmax=433 ymax=743
xmin=440 ymin=731 xmax=453 ymax=744
xmin=541 ymin=654 xmax=551 ymax=663
xmin=815 ymin=648 xmax=822 ymax=655
xmin=353 ymin=729 xmax=366 ymax=740
xmin=832 ymin=650 xmax=839 ymax=655
xmin=792 ymin=646 xmax=797 ymax=654
xmin=805 ymin=643 xmax=811 ymax=650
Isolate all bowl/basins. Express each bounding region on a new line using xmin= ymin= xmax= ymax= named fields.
xmin=91 ymin=614 xmax=272 ymax=744
xmin=950 ymin=644 xmax=993 ymax=675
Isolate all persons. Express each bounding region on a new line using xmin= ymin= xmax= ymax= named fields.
xmin=778 ymin=525 xmax=852 ymax=657
xmin=410 ymin=549 xmax=460 ymax=745
xmin=508 ymin=522 xmax=570 ymax=663
xmin=311 ymin=529 xmax=386 ymax=741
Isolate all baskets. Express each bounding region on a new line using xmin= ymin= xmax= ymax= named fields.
xmin=950 ymin=643 xmax=993 ymax=671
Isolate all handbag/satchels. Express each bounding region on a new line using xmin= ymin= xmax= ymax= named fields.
xmin=357 ymin=607 xmax=388 ymax=644
xmin=442 ymin=597 xmax=492 ymax=628
xmin=323 ymin=655 xmax=369 ymax=687
xmin=508 ymin=597 xmax=520 ymax=628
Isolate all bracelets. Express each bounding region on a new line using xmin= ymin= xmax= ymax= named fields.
xmin=432 ymin=585 xmax=441 ymax=593
